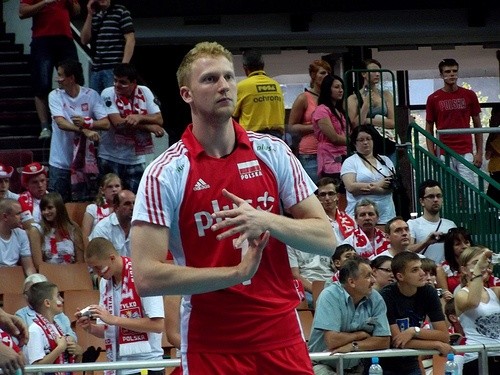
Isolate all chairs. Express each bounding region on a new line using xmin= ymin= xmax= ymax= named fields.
xmin=0 ymin=5 xmax=326 ymax=375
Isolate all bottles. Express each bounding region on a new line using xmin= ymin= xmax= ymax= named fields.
xmin=368 ymin=356 xmax=383 ymax=375
xmin=444 ymin=354 xmax=458 ymax=375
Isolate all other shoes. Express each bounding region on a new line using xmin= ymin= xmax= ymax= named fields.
xmin=39 ymin=128 xmax=51 ymax=140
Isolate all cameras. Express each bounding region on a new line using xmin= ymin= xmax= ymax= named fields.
xmin=489 ymin=253 xmax=500 ymax=263
xmin=387 ymin=175 xmax=400 ymax=191
xmin=78 ymin=305 xmax=94 ymax=319
xmin=438 ymin=233 xmax=447 ymax=242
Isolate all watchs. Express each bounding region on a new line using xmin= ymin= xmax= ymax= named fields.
xmin=414 ymin=327 xmax=421 ymax=336
xmin=352 ymin=342 xmax=359 ymax=351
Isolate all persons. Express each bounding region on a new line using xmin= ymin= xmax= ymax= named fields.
xmin=340 ymin=127 xmax=397 ymax=225
xmin=0 ymin=0 xmax=185 ymax=374
xmin=370 ymin=255 xmax=395 ymax=293
xmin=426 ymin=59 xmax=484 ymax=208
xmin=408 ymin=180 xmax=500 ymax=375
xmin=288 ymin=60 xmax=331 ymax=180
xmin=487 ymin=103 xmax=500 ymax=203
xmin=284 ymin=177 xmax=389 ymax=306
xmin=371 ymin=215 xmax=426 ymax=258
xmin=130 ymin=42 xmax=337 ymax=375
xmin=380 ymin=251 xmax=456 ymax=375
xmin=347 ymin=58 xmax=397 ymax=169
xmin=307 ymin=255 xmax=391 ymax=375
xmin=234 ymin=50 xmax=284 ymax=140
xmin=313 ymin=74 xmax=353 ymax=181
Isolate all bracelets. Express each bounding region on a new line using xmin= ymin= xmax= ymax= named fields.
xmin=471 ymin=275 xmax=482 ymax=280
xmin=79 ymin=127 xmax=83 ymax=133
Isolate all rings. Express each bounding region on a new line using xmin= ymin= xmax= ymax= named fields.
xmin=159 ymin=132 xmax=161 ymax=133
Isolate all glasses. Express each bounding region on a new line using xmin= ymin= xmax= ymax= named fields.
xmin=375 ymin=267 xmax=393 ymax=273
xmin=315 ymin=191 xmax=338 ymax=197
xmin=354 ymin=136 xmax=372 ymax=143
xmin=422 ymin=193 xmax=443 ymax=200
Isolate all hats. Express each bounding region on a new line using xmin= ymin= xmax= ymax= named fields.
xmin=0 ymin=163 xmax=14 ymax=179
xmin=16 ymin=163 xmax=44 ymax=174
